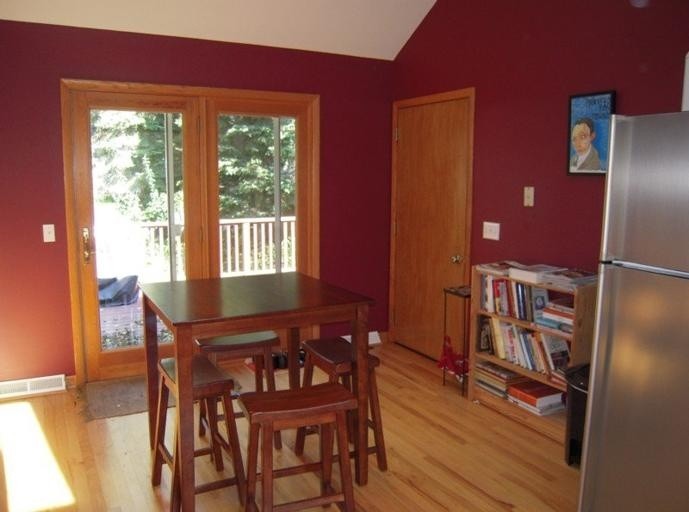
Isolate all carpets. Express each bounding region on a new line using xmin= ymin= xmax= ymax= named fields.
xmin=82 ymin=365 xmax=243 ymax=420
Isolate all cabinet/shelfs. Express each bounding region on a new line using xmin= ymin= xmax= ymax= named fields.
xmin=466 ymin=263 xmax=598 ymax=450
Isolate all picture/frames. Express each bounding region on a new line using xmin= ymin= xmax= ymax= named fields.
xmin=568 ymin=90 xmax=616 ymax=176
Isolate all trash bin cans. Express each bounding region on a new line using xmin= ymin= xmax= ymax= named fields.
xmin=565 ymin=364 xmax=591 ymax=470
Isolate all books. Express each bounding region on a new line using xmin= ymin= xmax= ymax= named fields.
xmin=474 ymin=260 xmax=600 ymax=417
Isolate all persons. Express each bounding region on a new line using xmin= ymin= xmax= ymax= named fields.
xmin=569 ymin=118 xmax=601 ymax=172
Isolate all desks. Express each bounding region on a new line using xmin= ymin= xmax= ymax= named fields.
xmin=140 ymin=270 xmax=376 ymax=512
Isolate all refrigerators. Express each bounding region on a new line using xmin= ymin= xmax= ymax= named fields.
xmin=573 ymin=111 xmax=688 ymax=511
